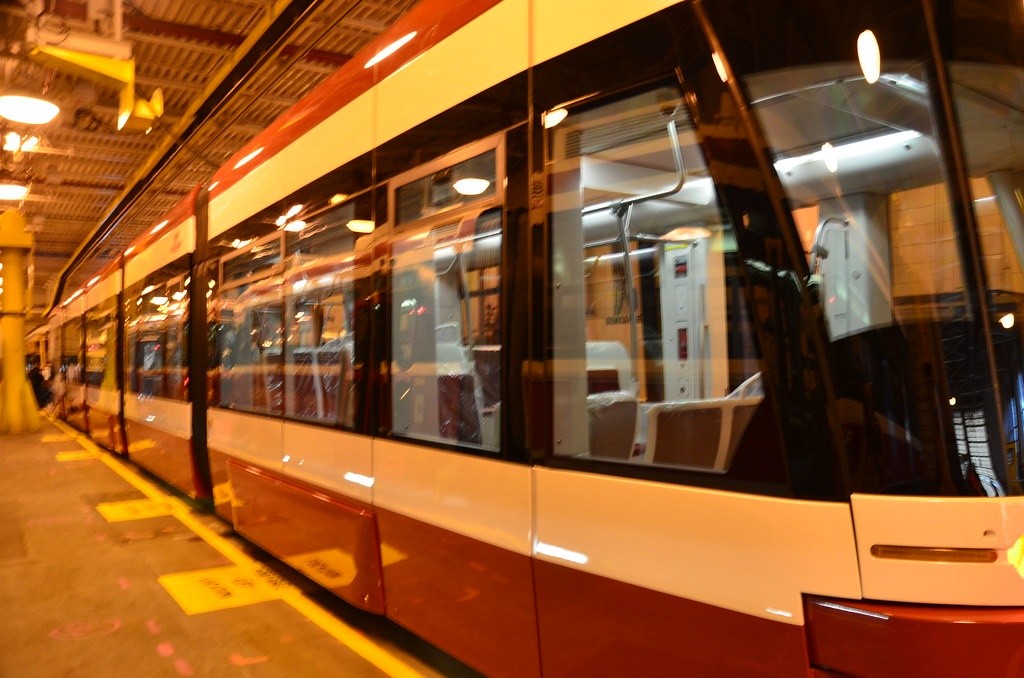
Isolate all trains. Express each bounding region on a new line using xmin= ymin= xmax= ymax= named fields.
xmin=26 ymin=0 xmax=1024 ymax=678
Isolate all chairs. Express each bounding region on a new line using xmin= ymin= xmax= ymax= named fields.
xmin=585 ymin=342 xmax=764 ymax=471
